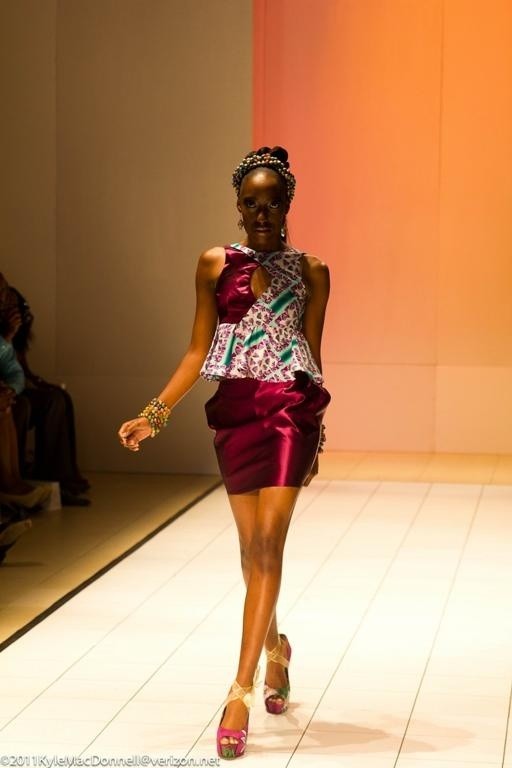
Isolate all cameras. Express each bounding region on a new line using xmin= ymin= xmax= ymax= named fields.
xmin=19 ymin=301 xmax=33 ymax=326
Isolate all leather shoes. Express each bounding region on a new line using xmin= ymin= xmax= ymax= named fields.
xmin=35 ymin=464 xmax=90 ymax=506
xmin=0 ymin=519 xmax=32 ymax=562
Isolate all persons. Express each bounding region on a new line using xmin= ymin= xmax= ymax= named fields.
xmin=118 ymin=146 xmax=329 ymax=760
xmin=0 ymin=271 xmax=91 ymax=567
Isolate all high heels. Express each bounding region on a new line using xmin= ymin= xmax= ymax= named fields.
xmin=217 ymin=680 xmax=252 ymax=760
xmin=264 ymin=634 xmax=292 ymax=714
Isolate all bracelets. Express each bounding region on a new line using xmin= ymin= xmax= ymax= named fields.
xmin=318 ymin=425 xmax=326 ymax=453
xmin=136 ymin=397 xmax=171 ymax=439
